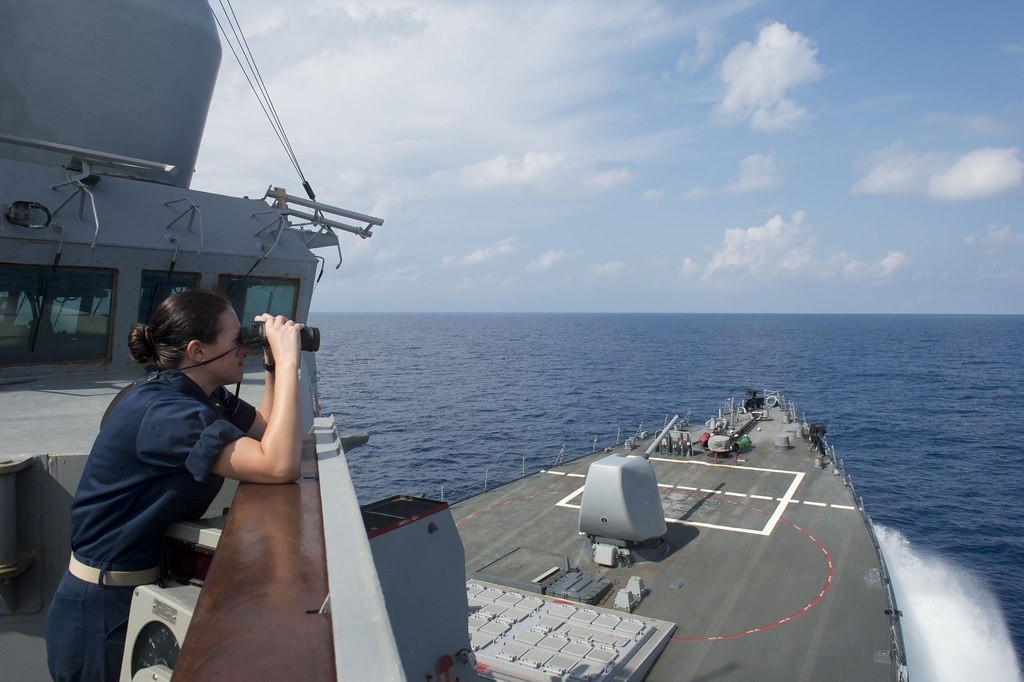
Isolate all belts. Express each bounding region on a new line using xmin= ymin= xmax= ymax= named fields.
xmin=68 ymin=551 xmax=160 ymax=586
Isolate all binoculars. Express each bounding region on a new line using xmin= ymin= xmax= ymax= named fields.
xmin=238 ymin=320 xmax=321 ymax=352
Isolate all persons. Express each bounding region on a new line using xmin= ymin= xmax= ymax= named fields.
xmin=45 ymin=290 xmax=306 ymax=682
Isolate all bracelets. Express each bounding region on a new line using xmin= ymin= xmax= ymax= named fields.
xmin=263 ymin=359 xmax=275 ymax=371
